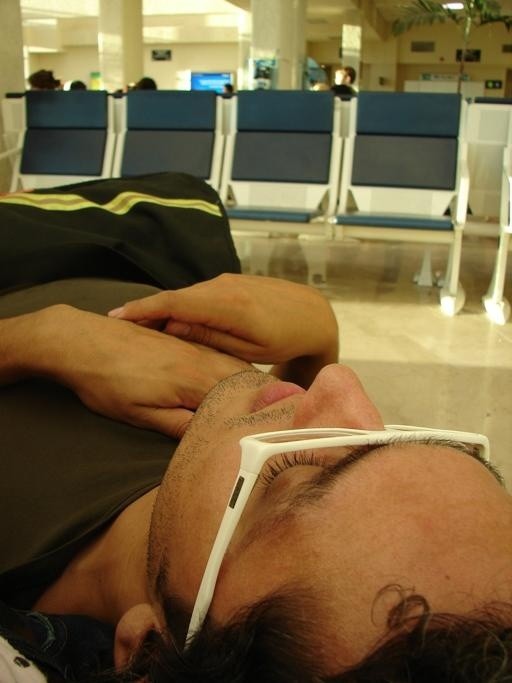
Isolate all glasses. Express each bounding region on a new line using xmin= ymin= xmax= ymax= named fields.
xmin=183 ymin=419 xmax=493 ymax=668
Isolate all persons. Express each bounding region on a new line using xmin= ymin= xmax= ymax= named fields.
xmin=70 ymin=81 xmax=87 ymax=91
xmin=311 ymin=66 xmax=356 ymax=93
xmin=1 ymin=166 xmax=510 ymax=680
xmin=135 ymin=77 xmax=157 ymax=90
xmin=224 ymin=83 xmax=234 ymax=93
xmin=28 ymin=69 xmax=60 ymax=89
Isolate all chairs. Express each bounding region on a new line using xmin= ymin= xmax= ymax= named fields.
xmin=332 ymin=90 xmax=459 ymax=236
xmin=8 ymin=91 xmax=115 ymax=192
xmin=216 ymin=90 xmax=337 ymax=223
xmin=439 ymin=95 xmax=512 ymax=327
xmin=111 ymin=90 xmax=226 ymax=194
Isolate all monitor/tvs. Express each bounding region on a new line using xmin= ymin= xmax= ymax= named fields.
xmin=189 ymin=70 xmax=234 ymax=92
